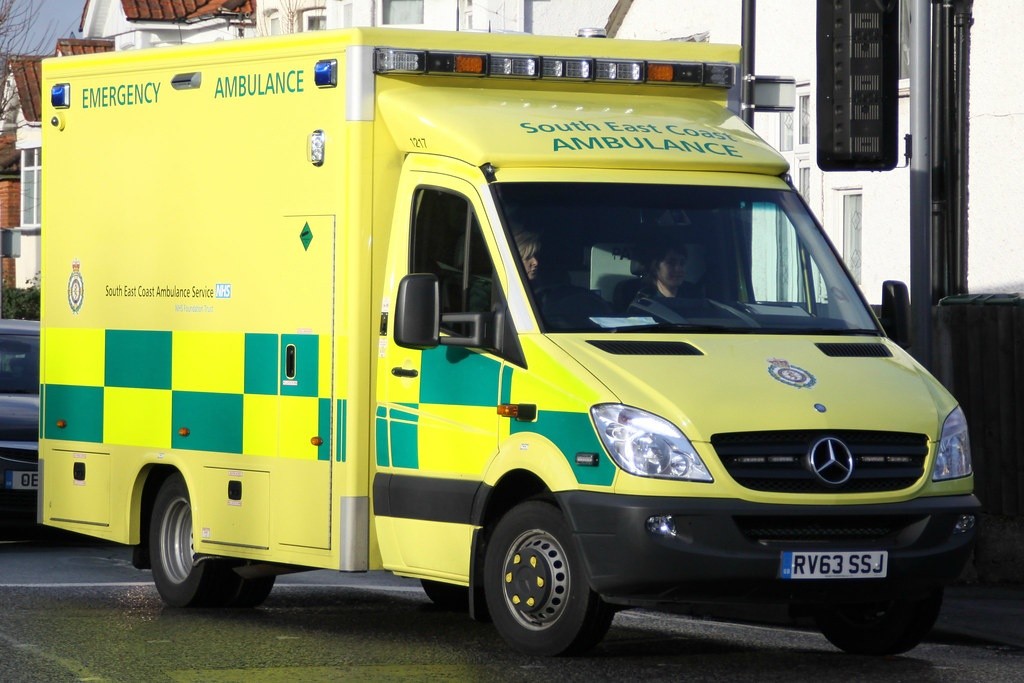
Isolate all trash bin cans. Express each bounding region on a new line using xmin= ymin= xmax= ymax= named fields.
xmin=938 ymin=294 xmax=1024 ymax=519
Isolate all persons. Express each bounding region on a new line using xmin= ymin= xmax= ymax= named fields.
xmin=632 ymin=233 xmax=708 ymax=315
xmin=470 ymin=221 xmax=583 ymax=332
xmin=9 ymin=358 xmax=33 ymax=382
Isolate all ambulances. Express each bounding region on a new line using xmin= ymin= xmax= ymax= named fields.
xmin=36 ymin=24 xmax=988 ymax=660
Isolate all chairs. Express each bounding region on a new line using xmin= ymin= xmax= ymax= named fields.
xmin=442 ymin=233 xmax=489 ymax=309
xmin=614 ymin=251 xmax=647 ymax=306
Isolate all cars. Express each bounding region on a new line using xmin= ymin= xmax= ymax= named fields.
xmin=0 ymin=318 xmax=41 ymax=529
